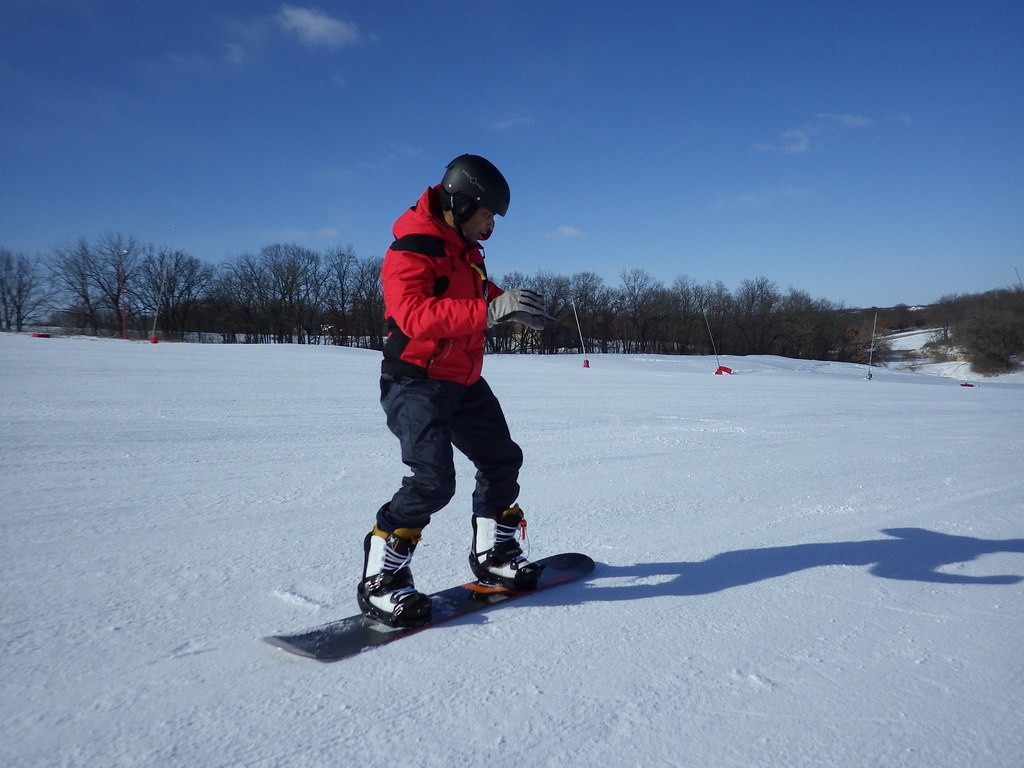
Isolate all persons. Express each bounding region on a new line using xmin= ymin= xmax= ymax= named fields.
xmin=358 ymin=154 xmax=546 ymax=627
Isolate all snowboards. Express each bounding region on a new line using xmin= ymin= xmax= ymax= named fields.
xmin=258 ymin=550 xmax=599 ymax=663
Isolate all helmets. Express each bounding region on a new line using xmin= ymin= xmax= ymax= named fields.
xmin=441 ymin=153 xmax=510 ymax=217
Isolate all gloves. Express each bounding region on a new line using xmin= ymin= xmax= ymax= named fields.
xmin=506 ymin=311 xmax=545 ymax=330
xmin=487 ymin=288 xmax=544 ymax=328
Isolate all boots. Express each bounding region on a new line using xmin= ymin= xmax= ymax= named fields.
xmin=357 ymin=528 xmax=432 ymax=628
xmin=469 ymin=503 xmax=543 ymax=589
